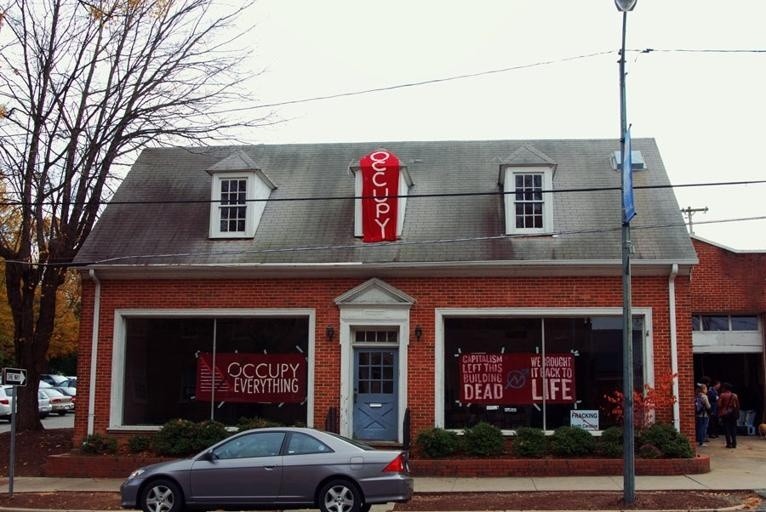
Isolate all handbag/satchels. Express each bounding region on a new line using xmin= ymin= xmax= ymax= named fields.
xmin=718 ymin=407 xmax=732 ymax=417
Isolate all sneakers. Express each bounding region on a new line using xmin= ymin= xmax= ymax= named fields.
xmin=709 ymin=433 xmax=719 ymax=439
xmin=697 ymin=442 xmax=708 ymax=447
xmin=725 ymin=444 xmax=737 ymax=448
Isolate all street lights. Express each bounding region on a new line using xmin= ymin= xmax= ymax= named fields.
xmin=614 ymin=1 xmax=639 ymax=505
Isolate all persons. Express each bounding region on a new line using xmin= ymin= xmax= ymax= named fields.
xmin=717 ymin=381 xmax=741 ymax=448
xmin=696 ymin=383 xmax=711 ymax=446
xmin=707 ymin=379 xmax=721 ymax=438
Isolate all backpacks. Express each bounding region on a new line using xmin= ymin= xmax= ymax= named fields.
xmin=695 ymin=394 xmax=707 ymax=413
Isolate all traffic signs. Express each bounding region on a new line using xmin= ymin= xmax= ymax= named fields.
xmin=4 ymin=368 xmax=28 ymax=388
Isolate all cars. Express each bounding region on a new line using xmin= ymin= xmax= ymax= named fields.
xmin=120 ymin=426 xmax=413 ymax=512
xmin=0 ymin=375 xmax=76 ymax=423
